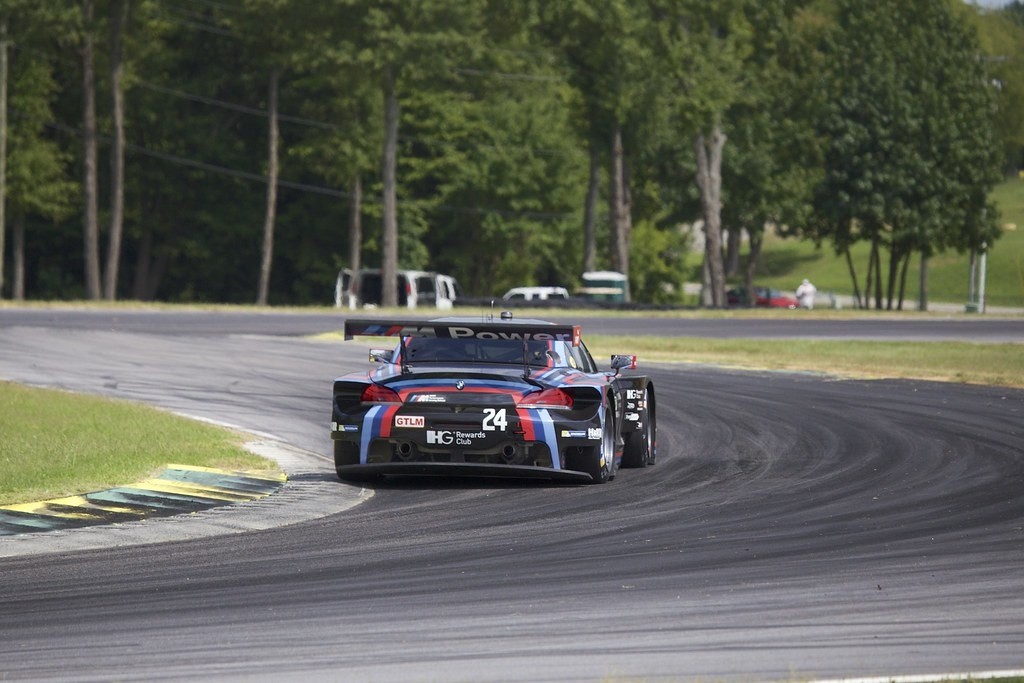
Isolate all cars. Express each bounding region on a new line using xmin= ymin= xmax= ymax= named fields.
xmin=727 ymin=286 xmax=800 ymax=310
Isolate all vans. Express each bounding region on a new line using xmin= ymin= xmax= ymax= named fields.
xmin=501 ymin=287 xmax=569 ymax=302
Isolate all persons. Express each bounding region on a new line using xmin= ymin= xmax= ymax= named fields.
xmin=796 ymin=278 xmax=819 ymax=309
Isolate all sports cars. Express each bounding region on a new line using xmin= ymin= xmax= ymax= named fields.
xmin=328 ymin=310 xmax=658 ymax=484
